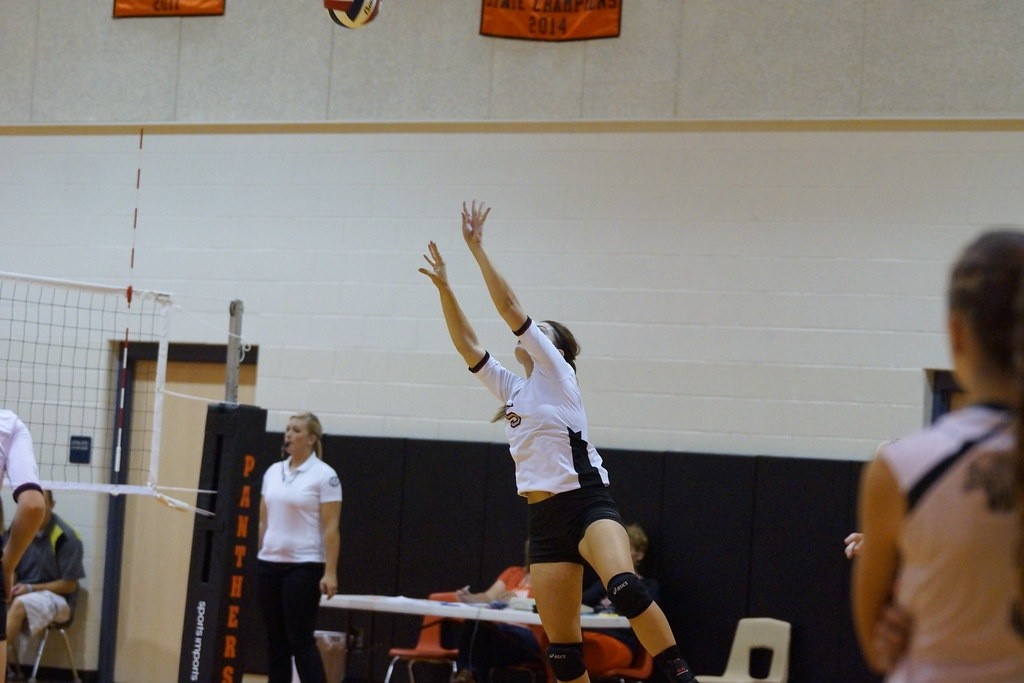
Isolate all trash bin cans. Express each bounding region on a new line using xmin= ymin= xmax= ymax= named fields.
xmin=315 ymin=631 xmax=353 ymax=682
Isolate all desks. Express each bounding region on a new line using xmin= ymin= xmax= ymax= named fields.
xmin=318 ymin=590 xmax=634 ymax=634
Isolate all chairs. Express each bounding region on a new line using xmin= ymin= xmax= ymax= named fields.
xmin=589 ymin=627 xmax=655 ymax=683
xmin=382 ymin=589 xmax=482 ymax=683
xmin=689 ymin=615 xmax=792 ymax=683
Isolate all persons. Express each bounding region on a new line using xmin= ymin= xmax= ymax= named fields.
xmin=851 ymin=230 xmax=1023 ymax=683
xmin=442 ymin=516 xmax=653 ymax=683
xmin=3 ymin=487 xmax=85 ymax=653
xmin=248 ymin=411 xmax=341 ymax=682
xmin=420 ymin=199 xmax=709 ymax=683
xmin=0 ymin=404 xmax=46 ymax=681
